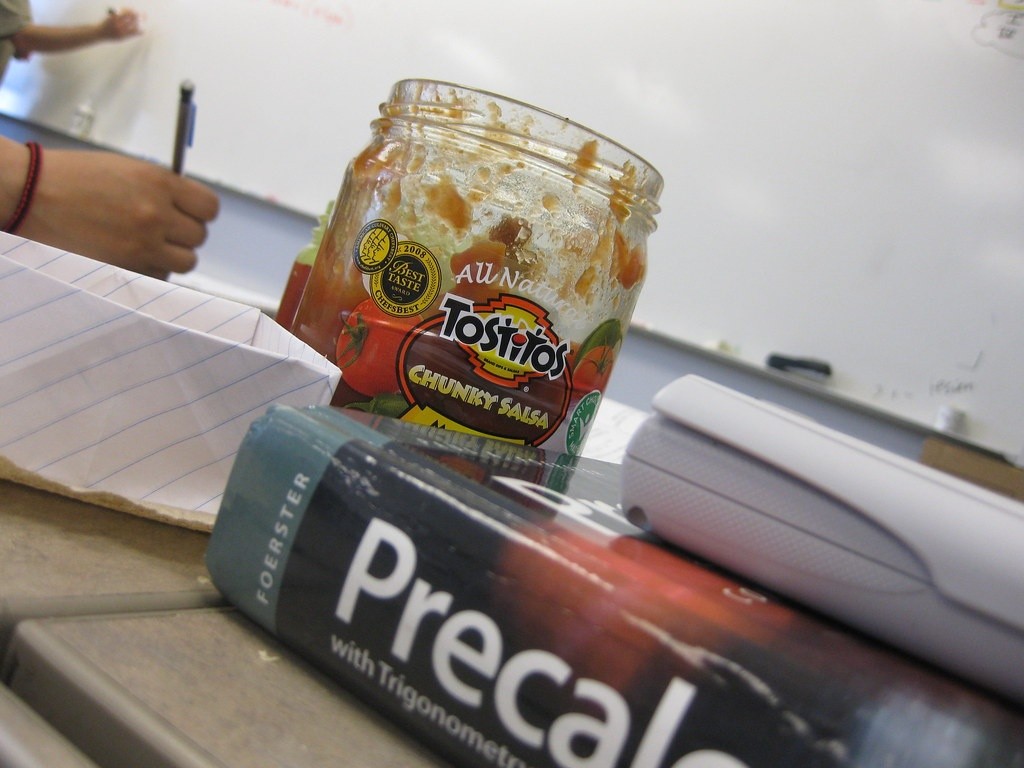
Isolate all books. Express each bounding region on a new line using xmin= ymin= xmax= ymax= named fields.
xmin=204 ymin=401 xmax=1024 ymax=766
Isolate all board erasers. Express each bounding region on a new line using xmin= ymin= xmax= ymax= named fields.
xmin=765 ymin=350 xmax=834 ymax=384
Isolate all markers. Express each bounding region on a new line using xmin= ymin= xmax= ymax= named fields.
xmin=109 ymin=8 xmax=118 ymax=16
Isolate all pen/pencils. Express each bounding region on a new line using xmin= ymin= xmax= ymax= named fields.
xmin=172 ymin=79 xmax=198 ymax=174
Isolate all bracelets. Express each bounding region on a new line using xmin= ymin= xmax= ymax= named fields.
xmin=0 ymin=140 xmax=42 ymax=236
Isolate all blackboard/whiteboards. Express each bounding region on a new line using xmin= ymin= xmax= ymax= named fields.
xmin=0 ymin=0 xmax=1023 ymax=470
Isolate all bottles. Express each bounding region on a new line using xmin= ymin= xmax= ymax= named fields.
xmin=281 ymin=199 xmax=330 ymax=321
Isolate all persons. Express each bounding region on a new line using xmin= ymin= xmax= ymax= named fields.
xmin=0 ymin=1 xmax=144 ymax=86
xmin=0 ymin=133 xmax=220 ymax=284
